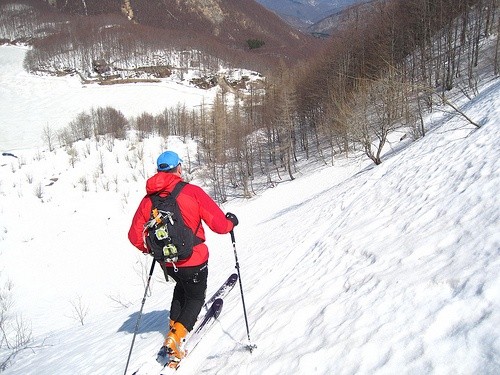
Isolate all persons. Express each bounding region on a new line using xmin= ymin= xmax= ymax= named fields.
xmin=128 ymin=151 xmax=239 ymax=361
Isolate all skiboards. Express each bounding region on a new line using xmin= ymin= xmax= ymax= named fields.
xmin=156 ymin=273 xmax=238 ymax=375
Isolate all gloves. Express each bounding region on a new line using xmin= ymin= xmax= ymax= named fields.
xmin=225 ymin=212 xmax=238 ymax=226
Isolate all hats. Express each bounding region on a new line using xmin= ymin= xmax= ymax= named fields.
xmin=157 ymin=151 xmax=183 ymax=170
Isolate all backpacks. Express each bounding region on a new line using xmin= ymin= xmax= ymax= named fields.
xmin=142 ymin=181 xmax=203 ymax=263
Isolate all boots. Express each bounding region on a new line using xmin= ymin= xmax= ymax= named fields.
xmin=158 ymin=320 xmax=188 ymax=360
